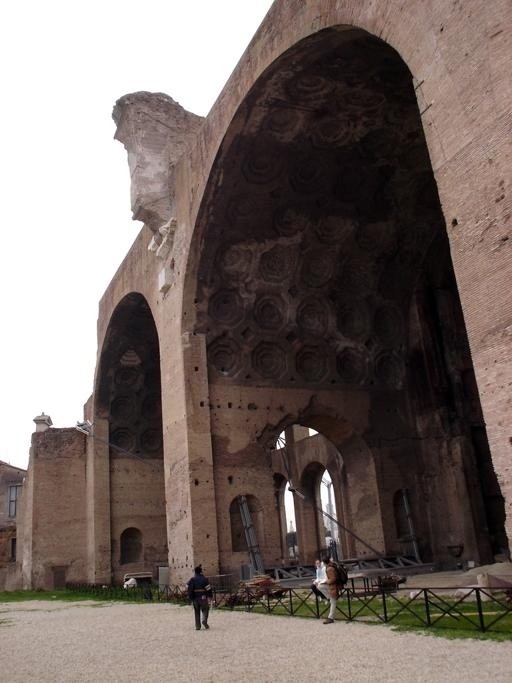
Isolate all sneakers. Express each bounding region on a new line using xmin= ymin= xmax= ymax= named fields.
xmin=202 ymin=620 xmax=209 ymax=629
xmin=323 ymin=619 xmax=333 ymax=624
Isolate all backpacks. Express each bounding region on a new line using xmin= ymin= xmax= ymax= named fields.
xmin=326 ymin=562 xmax=348 ymax=584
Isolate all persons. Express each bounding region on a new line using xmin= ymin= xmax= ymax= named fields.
xmin=310 ymin=559 xmax=330 ymax=606
xmin=322 ymin=555 xmax=340 ymax=624
xmin=188 ymin=566 xmax=213 ymax=630
